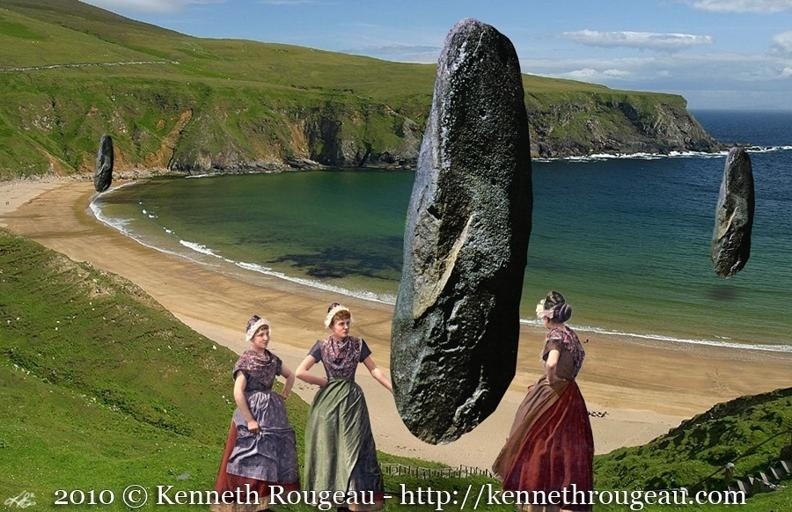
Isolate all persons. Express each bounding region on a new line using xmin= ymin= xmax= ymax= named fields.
xmin=293 ymin=302 xmax=394 ymax=511
xmin=489 ymin=289 xmax=597 ymax=512
xmin=212 ymin=315 xmax=302 ymax=511
